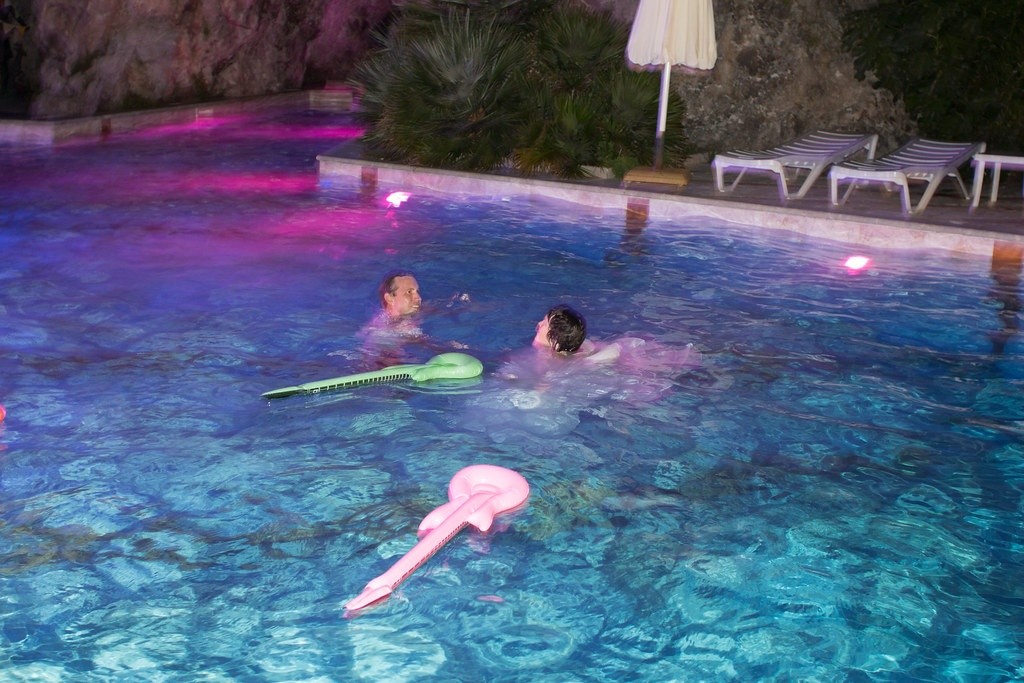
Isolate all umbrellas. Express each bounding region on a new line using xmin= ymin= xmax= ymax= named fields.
xmin=626 ymin=0 xmax=718 ymax=169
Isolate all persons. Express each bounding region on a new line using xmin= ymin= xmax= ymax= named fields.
xmin=379 ymin=269 xmax=422 ymax=315
xmin=534 ymin=304 xmax=587 ymax=352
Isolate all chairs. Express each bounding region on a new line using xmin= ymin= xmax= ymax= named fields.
xmin=829 ymin=137 xmax=986 ymax=215
xmin=712 ymin=129 xmax=879 ymax=199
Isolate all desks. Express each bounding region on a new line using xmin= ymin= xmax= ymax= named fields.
xmin=969 ymin=152 xmax=1024 ymax=207
